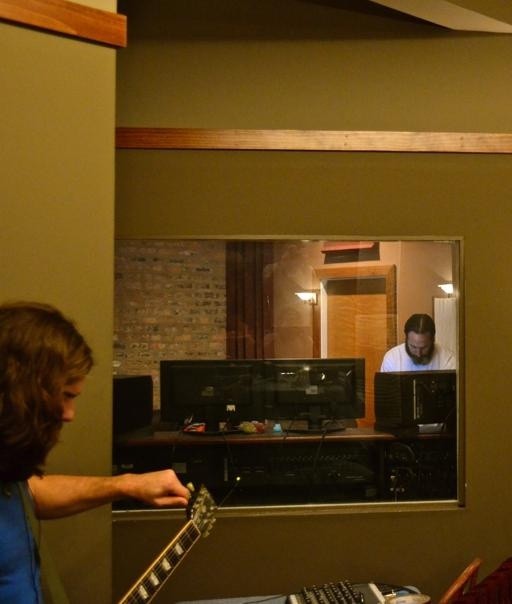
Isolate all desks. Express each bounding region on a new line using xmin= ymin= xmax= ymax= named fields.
xmin=120 ymin=417 xmax=459 ymax=500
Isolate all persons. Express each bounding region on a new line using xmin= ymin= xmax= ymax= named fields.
xmin=0 ymin=297 xmax=197 ymax=604
xmin=379 ymin=312 xmax=458 ymax=371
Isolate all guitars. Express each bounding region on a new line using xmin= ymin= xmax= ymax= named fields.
xmin=117 ymin=480 xmax=219 ymax=604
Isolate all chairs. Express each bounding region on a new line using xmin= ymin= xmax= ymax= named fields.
xmin=438 ymin=558 xmax=512 ymax=604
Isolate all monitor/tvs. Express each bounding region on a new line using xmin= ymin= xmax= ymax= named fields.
xmin=265 ymin=359 xmax=365 ymax=432
xmin=159 ymin=359 xmax=264 ymax=435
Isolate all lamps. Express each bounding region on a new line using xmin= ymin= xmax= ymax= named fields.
xmin=294 ymin=292 xmax=317 ymax=306
xmin=436 ymin=284 xmax=453 ymax=297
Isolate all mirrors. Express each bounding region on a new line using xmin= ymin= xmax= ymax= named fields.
xmin=110 ymin=233 xmax=466 ymax=522
